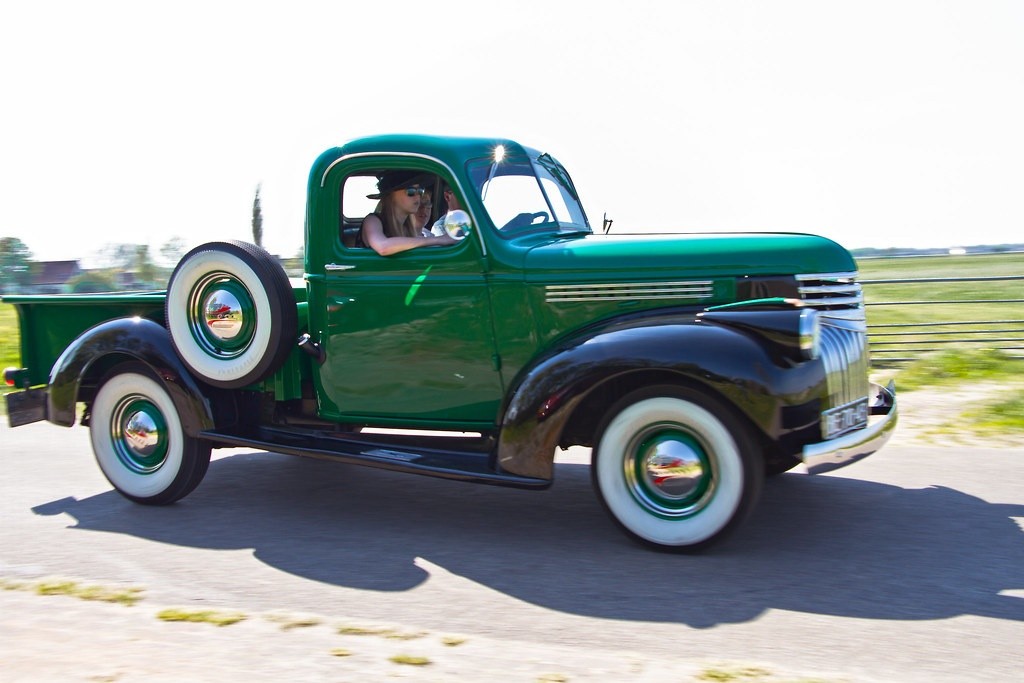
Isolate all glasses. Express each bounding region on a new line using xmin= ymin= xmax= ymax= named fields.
xmin=406 ymin=186 xmax=425 ymax=197
xmin=419 ymin=200 xmax=433 ymax=209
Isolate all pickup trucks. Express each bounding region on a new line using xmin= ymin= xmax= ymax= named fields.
xmin=0 ymin=135 xmax=898 ymax=556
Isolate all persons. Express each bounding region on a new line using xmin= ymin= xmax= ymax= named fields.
xmin=430 ymin=182 xmax=461 ymax=237
xmin=355 ymin=169 xmax=456 ymax=256
xmin=410 ymin=188 xmax=436 ymax=237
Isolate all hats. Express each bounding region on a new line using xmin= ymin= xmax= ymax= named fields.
xmin=443 ymin=183 xmax=452 ymax=192
xmin=366 ymin=170 xmax=425 ymax=199
